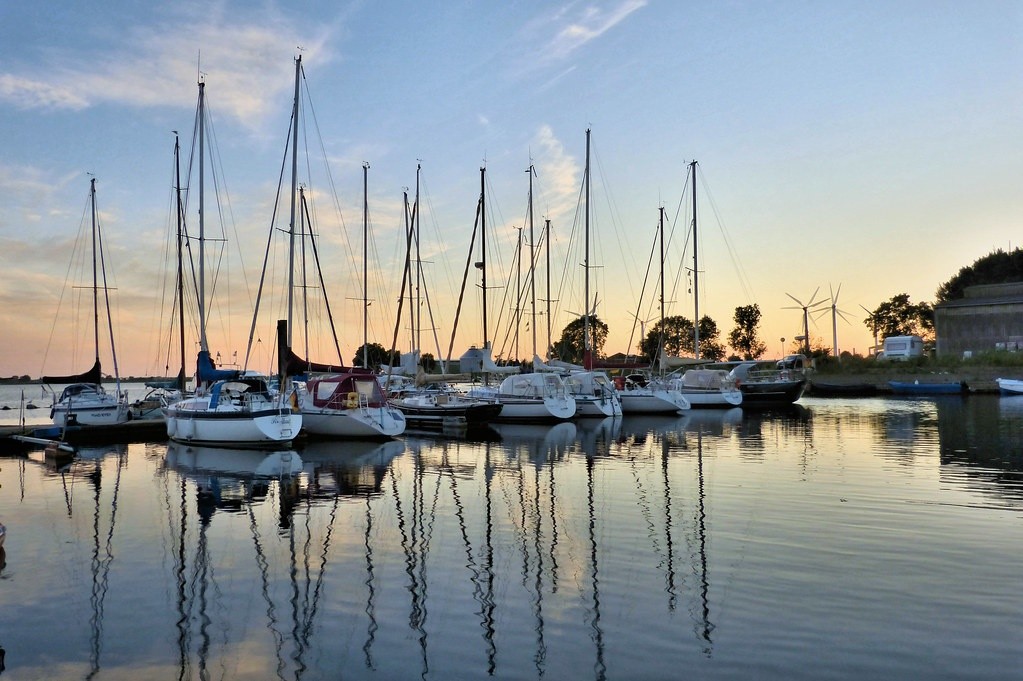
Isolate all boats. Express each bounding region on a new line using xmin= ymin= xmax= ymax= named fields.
xmin=994 ymin=377 xmax=1023 ymax=394
xmin=888 ymin=378 xmax=977 ymax=394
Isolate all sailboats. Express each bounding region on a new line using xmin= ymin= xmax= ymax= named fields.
xmin=2 ymin=47 xmax=878 ymax=452
xmin=158 ymin=408 xmax=741 ymax=681
